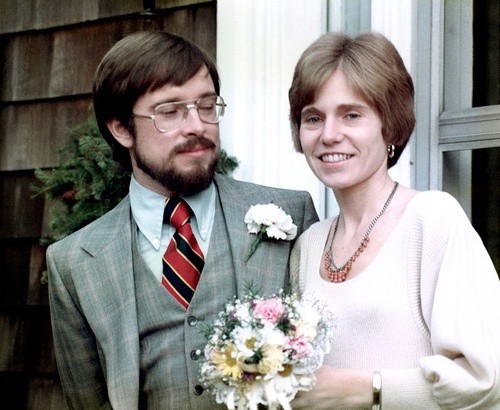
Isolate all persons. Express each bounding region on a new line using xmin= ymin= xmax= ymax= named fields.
xmin=271 ymin=32 xmax=500 ymax=409
xmin=45 ymin=29 xmax=321 ymax=410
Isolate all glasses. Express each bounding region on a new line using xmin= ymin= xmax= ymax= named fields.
xmin=131 ymin=95 xmax=227 ymax=132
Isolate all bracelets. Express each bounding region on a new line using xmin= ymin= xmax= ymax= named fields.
xmin=371 ymin=371 xmax=382 ymax=410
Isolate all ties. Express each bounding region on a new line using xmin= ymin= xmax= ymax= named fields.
xmin=162 ymin=195 xmax=204 ymax=308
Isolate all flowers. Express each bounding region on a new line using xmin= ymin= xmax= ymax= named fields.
xmin=244 ymin=204 xmax=297 ymax=264
xmin=198 ymin=279 xmax=336 ymax=410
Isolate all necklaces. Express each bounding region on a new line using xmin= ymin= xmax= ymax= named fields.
xmin=323 ymin=181 xmax=399 ymax=283
